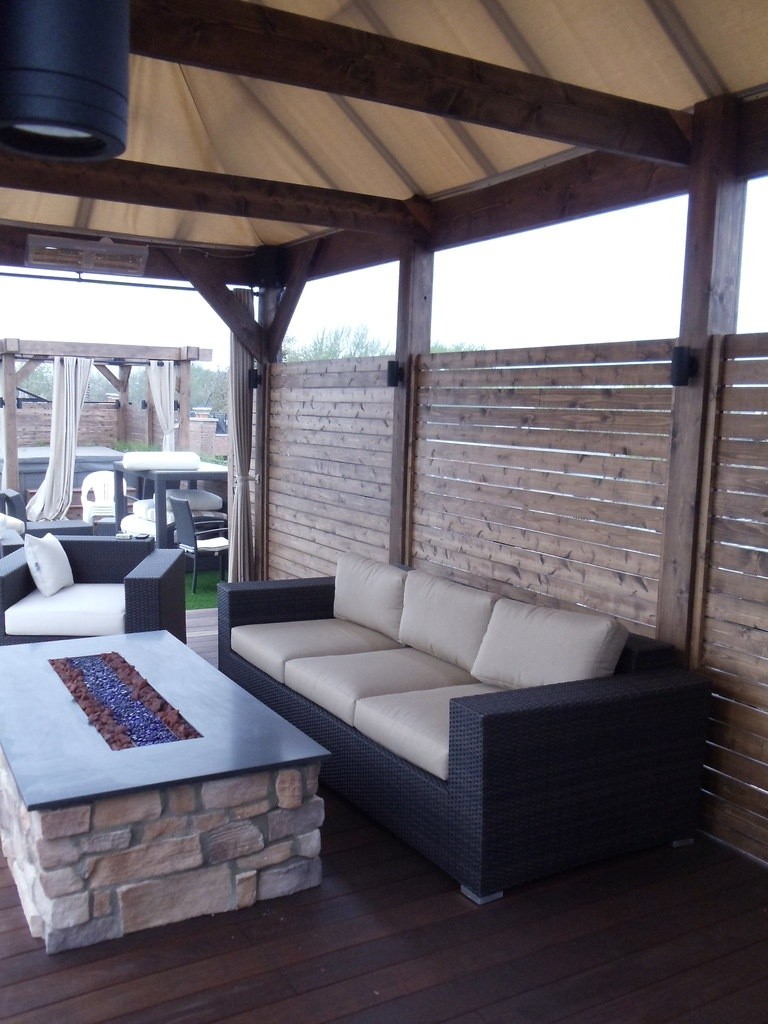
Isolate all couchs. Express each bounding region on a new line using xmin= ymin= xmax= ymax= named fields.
xmin=0 ymin=535 xmax=188 ymax=646
xmin=0 ymin=488 xmax=94 ymax=559
xmin=216 ymin=563 xmax=712 ymax=905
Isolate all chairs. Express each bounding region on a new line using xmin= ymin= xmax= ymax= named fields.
xmin=114 ymin=494 xmax=139 ymax=515
xmin=81 ymin=471 xmax=127 ymax=536
xmin=168 ymin=495 xmax=228 ymax=594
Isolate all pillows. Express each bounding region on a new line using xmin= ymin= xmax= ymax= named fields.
xmin=122 ymin=451 xmax=201 ymax=470
xmin=24 ymin=532 xmax=74 ymax=597
xmin=0 ymin=513 xmax=25 ymax=535
xmin=334 ymin=550 xmax=416 ymax=646
xmin=470 ymin=599 xmax=628 ymax=690
xmin=399 ymin=569 xmax=503 ymax=674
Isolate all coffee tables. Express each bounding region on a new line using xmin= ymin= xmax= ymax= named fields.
xmin=0 ymin=630 xmax=332 ymax=954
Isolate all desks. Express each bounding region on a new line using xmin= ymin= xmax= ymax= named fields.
xmin=113 ymin=461 xmax=229 ymax=573
